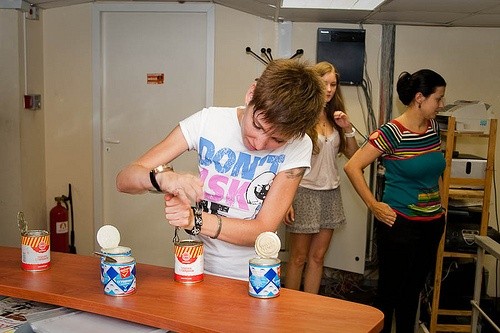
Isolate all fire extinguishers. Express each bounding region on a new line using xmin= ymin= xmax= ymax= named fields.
xmin=50 ymin=184 xmax=76 ymax=254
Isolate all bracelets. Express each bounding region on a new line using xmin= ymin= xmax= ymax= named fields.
xmin=344 ymin=128 xmax=356 ymax=137
xmin=210 ymin=213 xmax=222 ymax=240
xmin=183 ymin=206 xmax=203 ymax=236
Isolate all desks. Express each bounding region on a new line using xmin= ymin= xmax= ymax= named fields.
xmin=0 ymin=246 xmax=385 ymax=333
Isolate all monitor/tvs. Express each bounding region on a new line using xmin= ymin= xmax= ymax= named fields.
xmin=316 ymin=28 xmax=366 ymax=87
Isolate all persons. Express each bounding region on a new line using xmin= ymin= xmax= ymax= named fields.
xmin=115 ymin=58 xmax=324 ymax=281
xmin=343 ymin=69 xmax=446 ymax=333
xmin=282 ymin=60 xmax=358 ymax=293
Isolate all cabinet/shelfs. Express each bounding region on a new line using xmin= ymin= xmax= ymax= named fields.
xmin=418 ymin=116 xmax=499 ymax=333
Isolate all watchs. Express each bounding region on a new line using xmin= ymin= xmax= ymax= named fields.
xmin=149 ymin=164 xmax=174 ymax=193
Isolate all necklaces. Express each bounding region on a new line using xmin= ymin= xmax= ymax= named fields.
xmin=317 ymin=122 xmax=328 ymax=142
